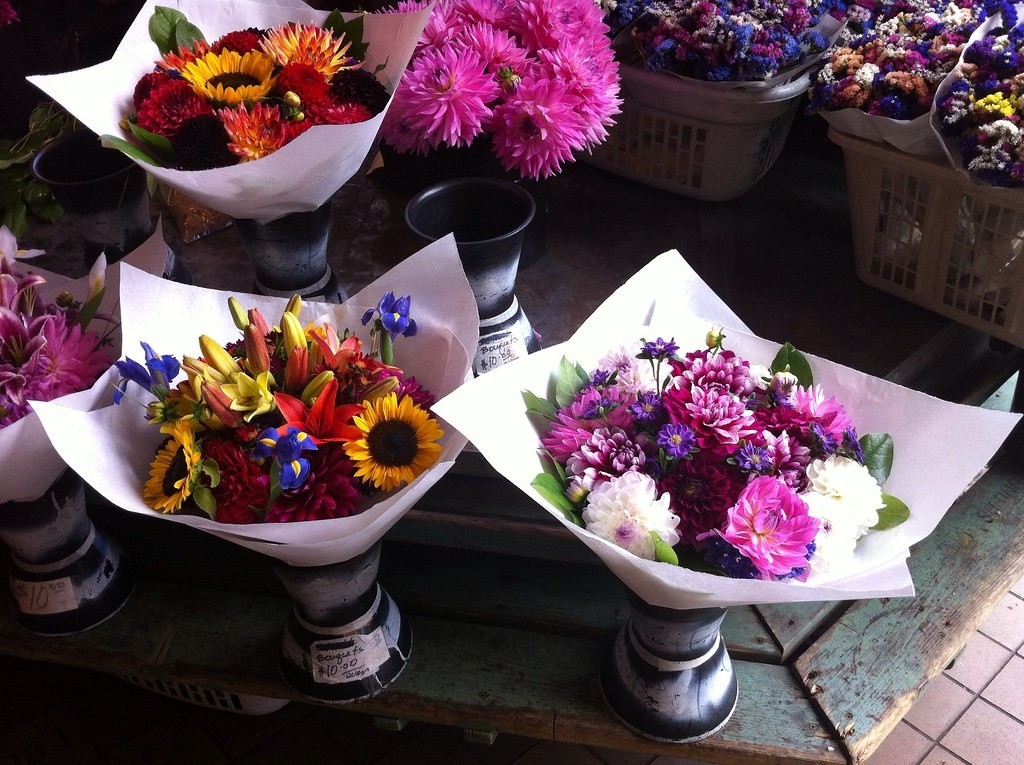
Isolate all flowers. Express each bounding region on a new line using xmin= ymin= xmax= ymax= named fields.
xmin=600 ymin=0 xmax=1024 ymax=187
xmin=372 ymin=0 xmax=624 ymax=180
xmin=519 ymin=326 xmax=912 ymax=586
xmin=121 ymin=7 xmax=390 ymax=168
xmin=109 ymin=293 xmax=442 ymax=519
xmin=0 ymin=225 xmax=118 ymax=430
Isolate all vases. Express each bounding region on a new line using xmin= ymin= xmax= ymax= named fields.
xmin=33 ymin=129 xmax=195 ymax=287
xmin=230 ymin=201 xmax=348 ymax=304
xmin=404 ymin=181 xmax=540 ymax=375
xmin=597 ymin=584 xmax=737 ymax=744
xmin=0 ymin=466 xmax=135 ymax=636
xmin=278 ymin=539 xmax=413 ymax=703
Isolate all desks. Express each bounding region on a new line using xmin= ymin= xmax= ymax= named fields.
xmin=0 ymin=0 xmax=1024 ymax=765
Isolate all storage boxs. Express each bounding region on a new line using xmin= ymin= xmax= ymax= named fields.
xmin=827 ymin=125 xmax=1024 ymax=349
xmin=572 ymin=65 xmax=802 ymax=201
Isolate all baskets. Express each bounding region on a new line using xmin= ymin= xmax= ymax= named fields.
xmin=827 ymin=119 xmax=1024 ymax=352
xmin=563 ymin=58 xmax=825 ymax=203
